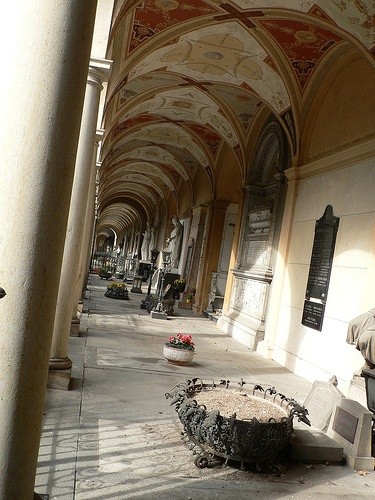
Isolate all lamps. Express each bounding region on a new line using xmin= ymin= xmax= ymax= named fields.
xmin=150 ymin=248 xmax=160 ymax=264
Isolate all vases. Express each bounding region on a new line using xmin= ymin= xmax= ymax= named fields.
xmin=162 ymin=345 xmax=195 ymax=366
xmin=176 ymin=285 xmax=185 ymax=292
xmin=104 ymin=287 xmax=130 ymax=301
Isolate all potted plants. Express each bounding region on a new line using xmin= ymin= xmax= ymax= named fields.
xmin=186 ymin=293 xmax=192 ymax=304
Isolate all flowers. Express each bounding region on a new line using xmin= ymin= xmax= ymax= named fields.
xmin=173 ymin=279 xmax=186 ymax=287
xmin=163 ymin=332 xmax=196 ymax=353
xmin=107 ymin=282 xmax=126 ymax=290
